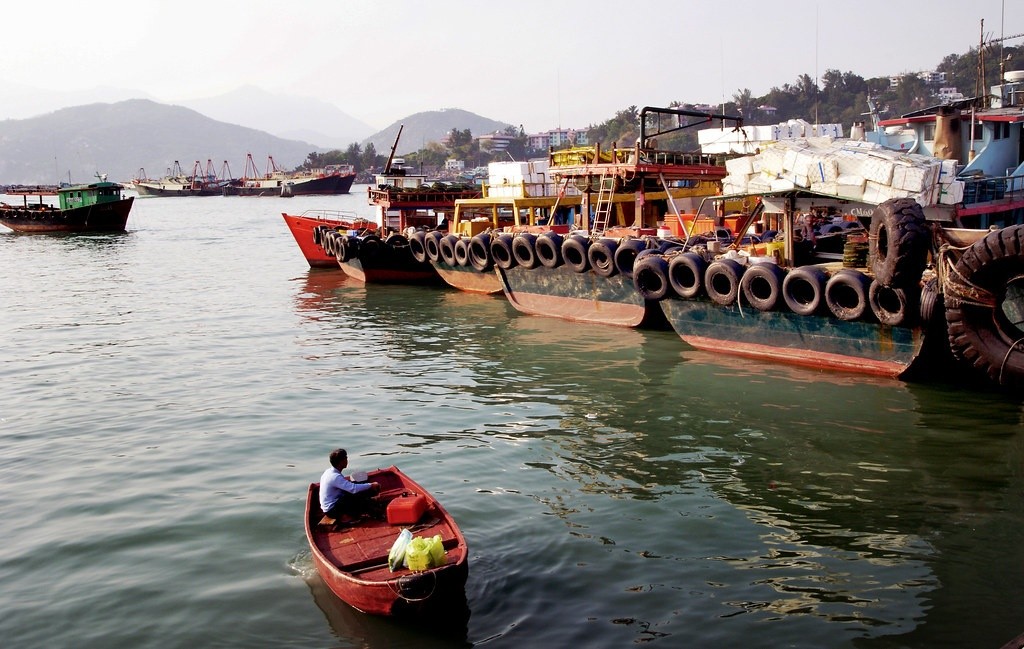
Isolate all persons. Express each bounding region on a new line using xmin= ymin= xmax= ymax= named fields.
xmin=319 ymin=448 xmax=381 ymax=516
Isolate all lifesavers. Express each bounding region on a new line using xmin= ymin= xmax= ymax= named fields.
xmin=554 ymin=172 xmax=562 ymax=184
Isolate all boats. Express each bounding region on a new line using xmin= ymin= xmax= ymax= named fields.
xmin=280 ymin=106 xmax=1024 ymax=389
xmin=304 ymin=466 xmax=468 ymax=617
xmin=0 ymin=194 xmax=135 ymax=235
xmin=6 ymin=188 xmax=58 ymax=195
xmin=132 ymin=153 xmax=357 ymax=196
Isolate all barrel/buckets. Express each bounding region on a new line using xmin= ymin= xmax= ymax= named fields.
xmin=656 ymin=225 xmax=672 ymax=238
xmin=843 ymin=232 xmax=869 ymax=267
xmin=956 ymin=168 xmax=1006 ymax=204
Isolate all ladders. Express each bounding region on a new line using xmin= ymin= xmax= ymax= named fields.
xmin=590 ymin=168 xmax=617 ymax=236
